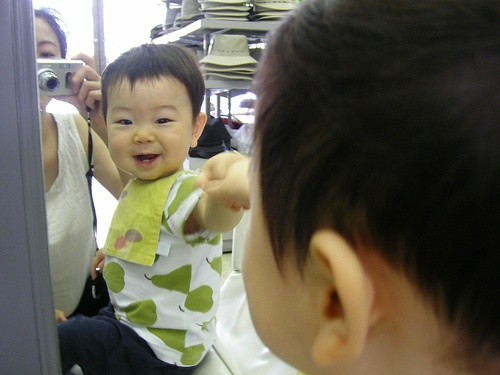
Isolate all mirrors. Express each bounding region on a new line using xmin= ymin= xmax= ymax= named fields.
xmin=31 ymin=0 xmax=305 ymax=375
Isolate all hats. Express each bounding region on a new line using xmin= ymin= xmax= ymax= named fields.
xmin=252 ymin=0 xmax=299 ymax=21
xmin=158 ymin=8 xmax=181 ymax=36
xmin=174 ymin=0 xmax=204 ymax=27
xmin=188 ymin=115 xmax=231 ymax=158
xmin=198 ymin=0 xmax=251 ymax=21
xmin=198 ymin=34 xmax=259 ymax=80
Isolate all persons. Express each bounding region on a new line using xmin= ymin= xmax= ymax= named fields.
xmin=210 ymin=0 xmax=500 ymax=375
xmin=34 ymin=9 xmax=133 ymax=322
xmin=56 ymin=42 xmax=256 ymax=375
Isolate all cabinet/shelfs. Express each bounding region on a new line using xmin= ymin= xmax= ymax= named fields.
xmin=152 ymin=18 xmax=275 ymax=272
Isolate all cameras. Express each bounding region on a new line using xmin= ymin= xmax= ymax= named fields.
xmin=36 ymin=58 xmax=86 ymax=97
